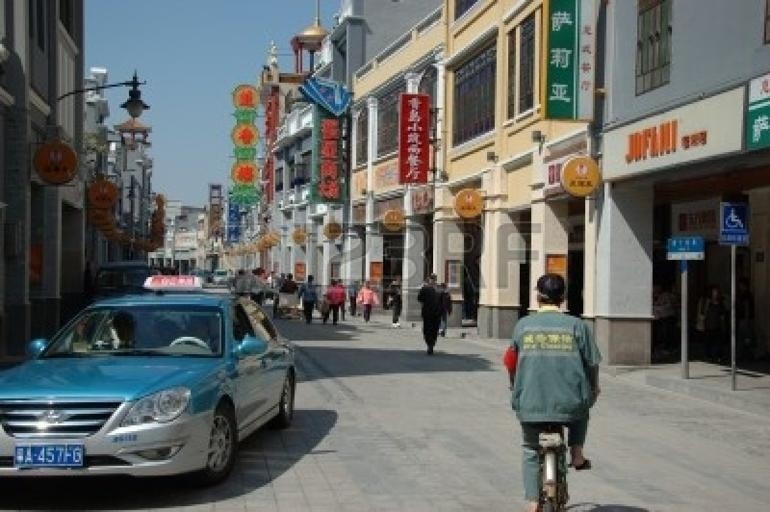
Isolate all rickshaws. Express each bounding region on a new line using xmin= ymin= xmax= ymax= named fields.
xmin=273 ymin=288 xmax=305 ymax=319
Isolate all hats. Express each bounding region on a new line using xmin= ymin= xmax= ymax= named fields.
xmin=536 ymin=273 xmax=564 ymax=297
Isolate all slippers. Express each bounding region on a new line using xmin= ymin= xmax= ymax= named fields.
xmin=577 ymin=458 xmax=589 ymax=471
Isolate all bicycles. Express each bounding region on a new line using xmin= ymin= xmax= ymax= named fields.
xmin=509 ymin=386 xmax=600 ymax=511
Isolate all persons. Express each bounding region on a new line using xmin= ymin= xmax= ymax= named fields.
xmin=417 ymin=272 xmax=453 ymax=354
xmin=653 ymin=275 xmax=754 ymax=345
xmin=233 ymin=266 xmax=403 ymax=327
xmin=504 ymin=273 xmax=601 ymax=512
xmin=93 ymin=312 xmax=216 ymax=354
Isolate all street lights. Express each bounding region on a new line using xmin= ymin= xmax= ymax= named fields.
xmin=55 ymin=70 xmax=151 ymax=120
xmin=171 ymin=215 xmax=188 ymax=265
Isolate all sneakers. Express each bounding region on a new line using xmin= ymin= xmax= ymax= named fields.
xmin=441 ymin=331 xmax=446 ymax=337
xmin=393 ymin=322 xmax=401 ymax=328
